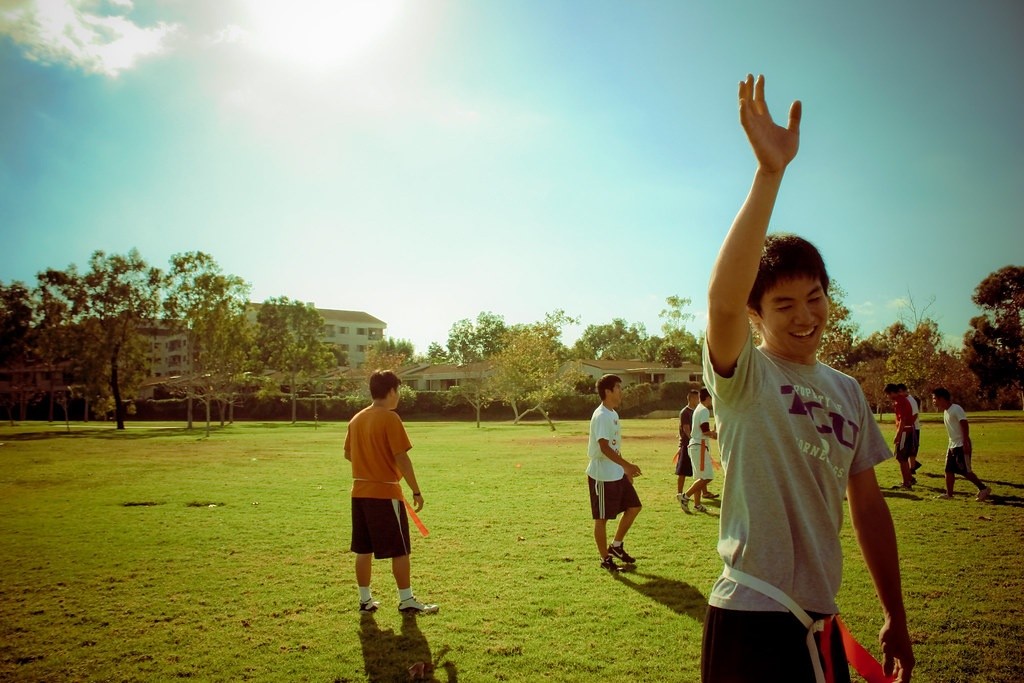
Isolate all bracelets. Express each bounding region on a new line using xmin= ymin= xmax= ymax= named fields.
xmin=412 ymin=492 xmax=422 ymax=497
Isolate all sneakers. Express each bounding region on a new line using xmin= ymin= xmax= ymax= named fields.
xmin=976 ymin=487 xmax=991 ymax=502
xmin=677 ymin=492 xmax=692 ymax=514
xmin=893 ymin=477 xmax=917 ymax=491
xmin=359 ymin=598 xmax=378 ymax=613
xmin=702 ymin=492 xmax=719 ymax=498
xmin=933 ymin=494 xmax=955 ymax=500
xmin=608 ymin=542 xmax=635 ymax=562
xmin=601 ymin=556 xmax=625 ymax=571
xmin=911 ymin=461 xmax=921 ymax=474
xmin=398 ymin=597 xmax=439 ymax=613
xmin=694 ymin=504 xmax=706 ymax=512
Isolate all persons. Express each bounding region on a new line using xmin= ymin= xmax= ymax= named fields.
xmin=894 ymin=382 xmax=923 ymax=475
xmin=342 ymin=369 xmax=441 ymax=615
xmin=675 ymin=385 xmax=717 ymax=516
xmin=584 ymin=373 xmax=644 ymax=572
xmin=674 ymin=389 xmax=720 ymax=500
xmin=882 ymin=383 xmax=917 ymax=490
xmin=696 ymin=69 xmax=918 ymax=683
xmin=929 ymin=386 xmax=992 ymax=502
